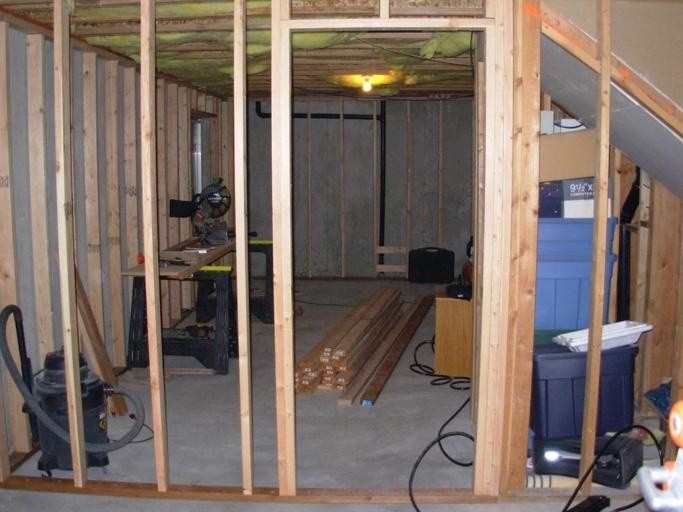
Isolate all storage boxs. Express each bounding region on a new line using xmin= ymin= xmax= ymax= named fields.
xmin=533 ymin=342 xmax=645 ymax=443
xmin=536 ymin=212 xmax=620 ymax=335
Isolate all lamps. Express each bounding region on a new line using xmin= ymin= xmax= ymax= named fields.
xmin=357 ymin=74 xmax=381 ymax=96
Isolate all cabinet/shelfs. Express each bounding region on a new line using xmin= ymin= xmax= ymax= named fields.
xmin=433 ymin=295 xmax=472 ymax=379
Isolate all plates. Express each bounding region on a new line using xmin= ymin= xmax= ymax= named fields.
xmin=551 ymin=320 xmax=653 ymax=352
